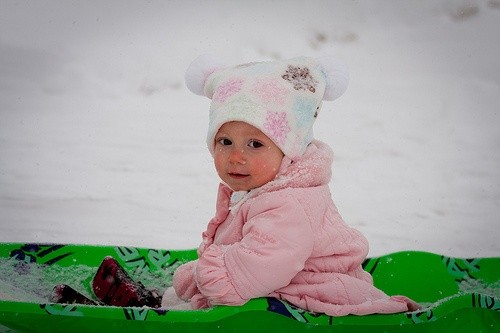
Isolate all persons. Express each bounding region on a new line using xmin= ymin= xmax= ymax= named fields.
xmin=48 ymin=52 xmax=410 ymax=317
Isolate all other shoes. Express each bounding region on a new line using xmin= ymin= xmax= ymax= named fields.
xmin=92 ymin=257 xmax=162 ymax=307
xmin=49 ymin=283 xmax=96 ymax=305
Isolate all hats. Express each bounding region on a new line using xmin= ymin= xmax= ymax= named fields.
xmin=186 ymin=51 xmax=328 ymax=165
